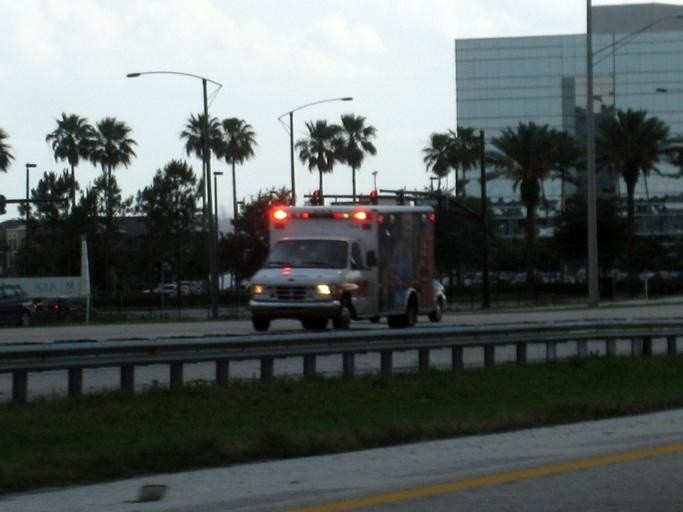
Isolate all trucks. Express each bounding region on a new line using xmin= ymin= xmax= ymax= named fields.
xmin=249 ymin=204 xmax=435 ymax=331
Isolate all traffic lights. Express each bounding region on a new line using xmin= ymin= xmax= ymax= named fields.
xmin=371 ymin=191 xmax=378 ymax=204
xmin=311 ymin=191 xmax=321 ymax=204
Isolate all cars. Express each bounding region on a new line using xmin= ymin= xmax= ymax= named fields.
xmin=369 ymin=280 xmax=446 ymax=322
xmin=463 ymin=268 xmax=585 ymax=285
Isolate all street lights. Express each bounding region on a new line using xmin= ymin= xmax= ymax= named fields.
xmin=277 ymin=97 xmax=352 ymax=207
xmin=25 ymin=162 xmax=37 ymax=243
xmin=128 ymin=71 xmax=223 ymax=322
xmin=586 ymin=0 xmax=682 ymax=304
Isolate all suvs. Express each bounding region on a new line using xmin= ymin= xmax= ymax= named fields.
xmin=0 ymin=284 xmax=74 ymax=326
